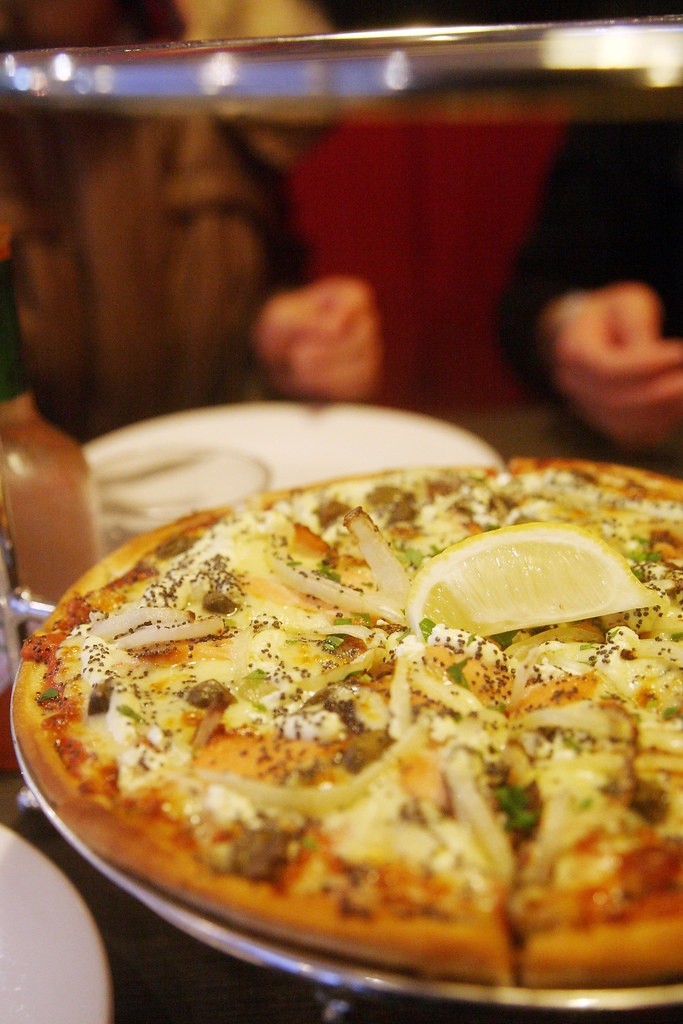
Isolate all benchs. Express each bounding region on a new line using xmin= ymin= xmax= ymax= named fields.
xmin=301 ymin=112 xmax=580 ymax=411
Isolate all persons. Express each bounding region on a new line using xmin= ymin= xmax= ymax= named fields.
xmin=496 ymin=118 xmax=683 ymax=473
xmin=0 ymin=0 xmax=384 ymax=440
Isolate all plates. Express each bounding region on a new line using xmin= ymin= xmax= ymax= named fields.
xmin=83 ymin=399 xmax=500 ymax=534
xmin=4 ymin=591 xmax=683 ymax=1014
xmin=3 ymin=824 xmax=112 ymax=1021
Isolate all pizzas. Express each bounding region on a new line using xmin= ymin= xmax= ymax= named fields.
xmin=9 ymin=463 xmax=683 ymax=985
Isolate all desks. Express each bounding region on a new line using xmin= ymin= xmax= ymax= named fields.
xmin=0 ymin=403 xmax=683 ymax=1024
